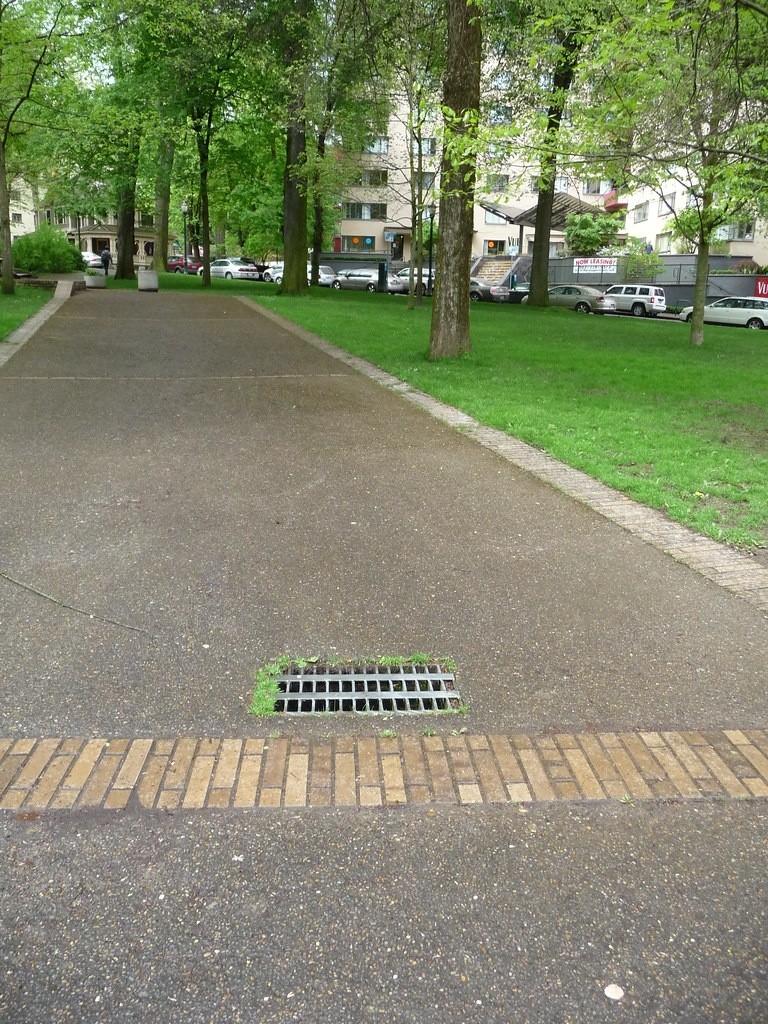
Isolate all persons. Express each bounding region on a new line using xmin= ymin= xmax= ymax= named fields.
xmin=101 ymin=246 xmax=115 ymax=277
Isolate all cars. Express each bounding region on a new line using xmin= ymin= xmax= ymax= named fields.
xmin=196 ymin=258 xmax=259 ymax=282
xmin=520 ymin=283 xmax=617 ymax=316
xmin=80 ymin=251 xmax=103 ymax=268
xmin=166 ymin=254 xmax=202 ymax=275
xmin=680 ymin=294 xmax=768 ymax=333
xmin=469 ymin=276 xmax=510 ymax=303
xmin=262 ymin=263 xmax=284 ymax=285
xmin=329 ymin=269 xmax=404 ymax=295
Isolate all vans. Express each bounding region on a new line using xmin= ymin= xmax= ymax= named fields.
xmin=601 ymin=284 xmax=667 ymax=318
xmin=306 ymin=265 xmax=337 ymax=288
xmin=394 ymin=267 xmax=435 ymax=296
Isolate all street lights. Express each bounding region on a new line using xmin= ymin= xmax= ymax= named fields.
xmin=180 ymin=202 xmax=189 ymax=274
